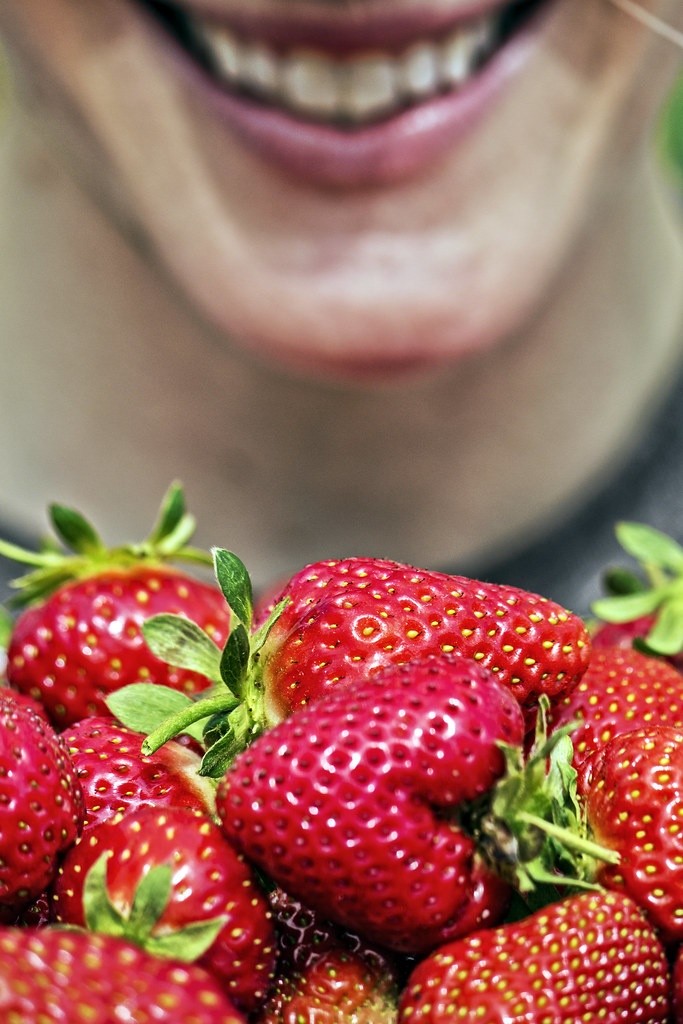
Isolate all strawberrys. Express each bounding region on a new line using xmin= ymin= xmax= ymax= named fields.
xmin=0 ymin=479 xmax=683 ymax=1024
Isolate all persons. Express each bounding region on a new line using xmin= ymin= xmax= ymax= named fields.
xmin=0 ymin=0 xmax=682 ymax=664
